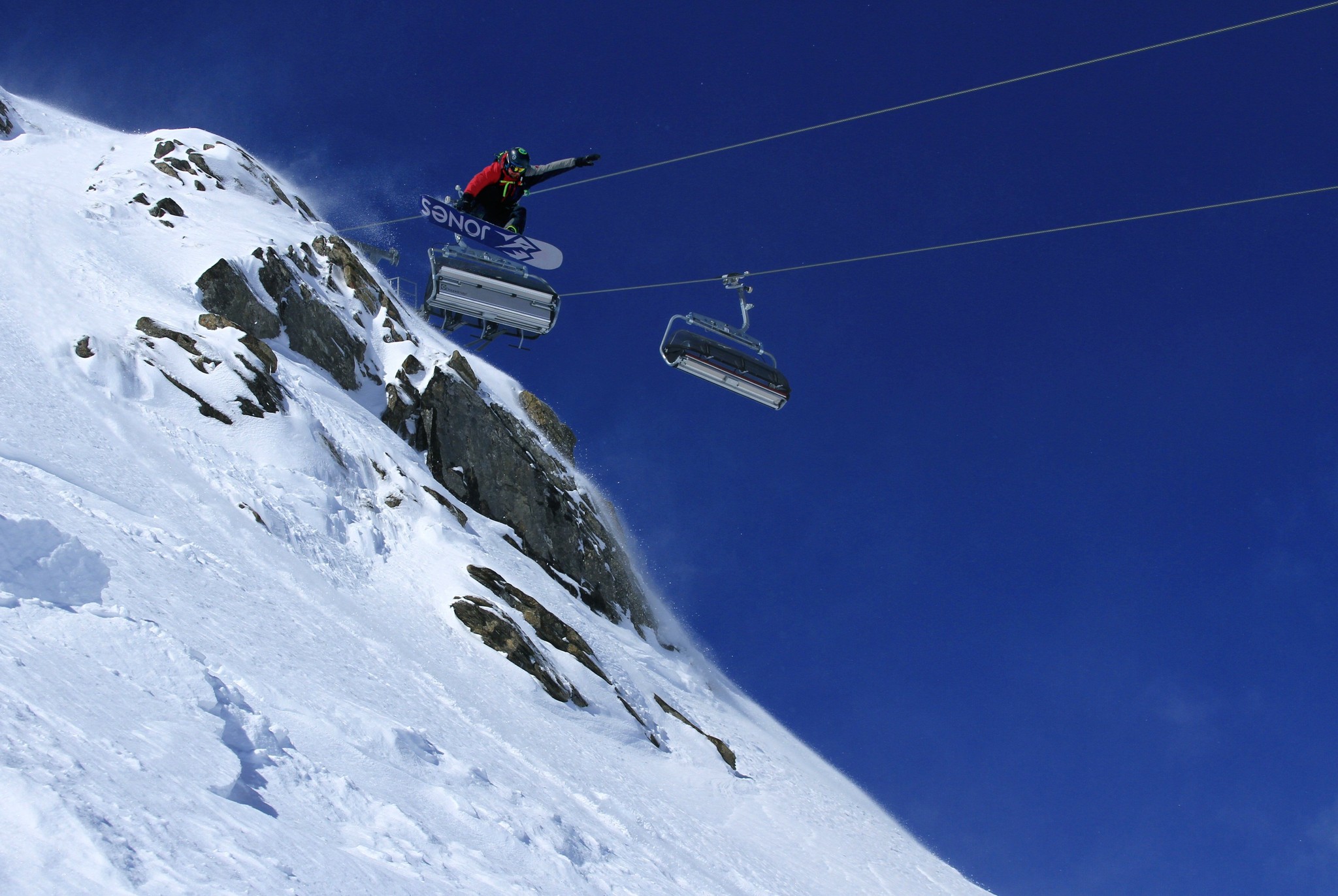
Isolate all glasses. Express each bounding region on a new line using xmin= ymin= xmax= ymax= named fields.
xmin=509 ymin=164 xmax=525 ymax=173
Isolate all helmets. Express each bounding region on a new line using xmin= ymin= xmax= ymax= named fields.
xmin=506 ymin=147 xmax=530 ymax=168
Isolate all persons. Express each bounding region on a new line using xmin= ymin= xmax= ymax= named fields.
xmin=453 ymin=147 xmax=602 ymax=234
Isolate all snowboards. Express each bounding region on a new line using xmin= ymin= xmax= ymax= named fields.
xmin=419 ymin=195 xmax=564 ymax=270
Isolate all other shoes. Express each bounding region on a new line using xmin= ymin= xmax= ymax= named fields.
xmin=505 ymin=226 xmax=517 ymax=233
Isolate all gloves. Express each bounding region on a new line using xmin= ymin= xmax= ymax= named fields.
xmin=575 ymin=154 xmax=600 ymax=168
xmin=457 ymin=192 xmax=474 ymax=213
